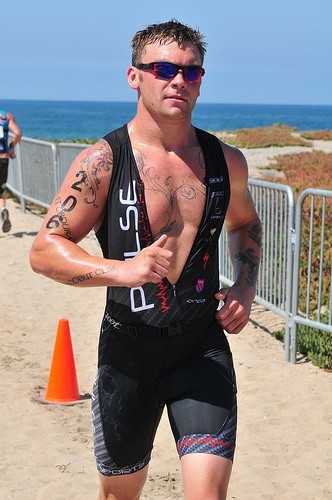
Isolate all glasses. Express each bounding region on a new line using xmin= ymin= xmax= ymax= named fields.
xmin=134 ymin=62 xmax=205 ymax=84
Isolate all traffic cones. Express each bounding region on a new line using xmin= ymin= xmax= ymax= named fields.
xmin=31 ymin=318 xmax=91 ymax=406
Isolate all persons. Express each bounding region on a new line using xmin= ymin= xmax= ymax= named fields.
xmin=29 ymin=18 xmax=262 ymax=500
xmin=0 ymin=110 xmax=22 ymax=233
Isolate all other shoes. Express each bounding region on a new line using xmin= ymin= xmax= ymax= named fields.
xmin=0 ymin=208 xmax=12 ymax=233
